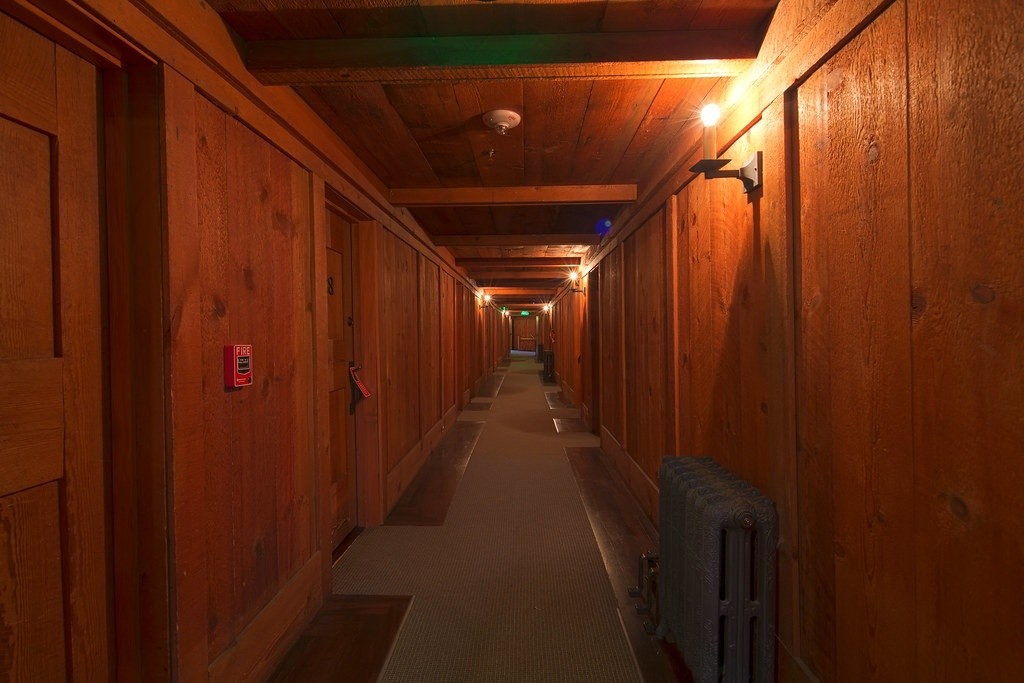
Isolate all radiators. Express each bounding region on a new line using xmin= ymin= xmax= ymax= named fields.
xmin=628 ymin=456 xmax=779 ymax=683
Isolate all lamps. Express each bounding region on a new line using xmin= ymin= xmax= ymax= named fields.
xmin=689 ymin=103 xmax=763 ymax=193
